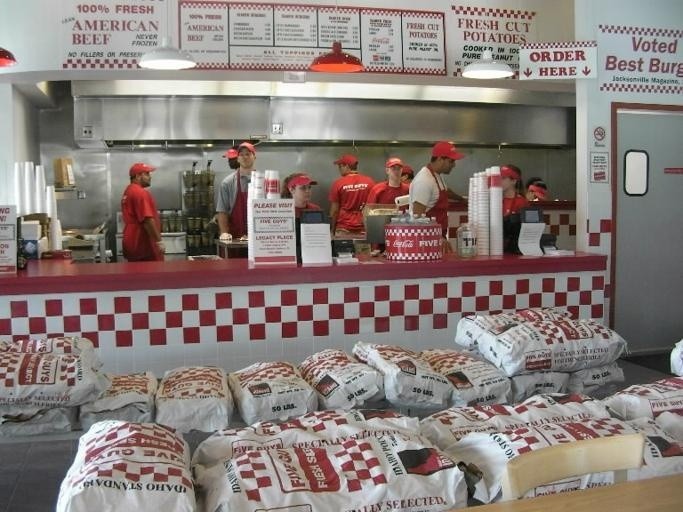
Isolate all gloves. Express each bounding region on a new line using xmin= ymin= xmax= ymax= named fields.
xmin=156 ymin=239 xmax=165 ymax=253
xmin=219 ymin=232 xmax=232 ymax=240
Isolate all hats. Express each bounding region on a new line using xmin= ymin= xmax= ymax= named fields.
xmin=333 ymin=154 xmax=358 ymax=167
xmin=239 ymin=142 xmax=256 ymax=153
xmin=431 ymin=141 xmax=464 ymax=160
xmin=384 ymin=157 xmax=403 ymax=167
xmin=287 ymin=175 xmax=317 ymax=191
xmin=501 ymin=166 xmax=520 ymax=180
xmin=402 ymin=166 xmax=413 ymax=174
xmin=128 ymin=162 xmax=156 ymax=177
xmin=222 ymin=148 xmax=237 ymax=159
xmin=528 ymin=185 xmax=547 ymax=200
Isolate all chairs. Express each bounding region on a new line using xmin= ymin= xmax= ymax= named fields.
xmin=503 ymin=433 xmax=644 ymax=501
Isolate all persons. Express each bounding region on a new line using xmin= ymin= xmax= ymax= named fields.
xmin=401 ymin=165 xmax=414 ymax=185
xmin=122 ymin=161 xmax=165 ymax=260
xmin=409 ymin=142 xmax=468 ymax=240
xmin=329 ymin=155 xmax=375 ymax=238
xmin=501 ymin=165 xmax=532 ymax=217
xmin=216 ymin=142 xmax=256 ymax=259
xmin=367 ymin=157 xmax=409 ymax=254
xmin=222 ymin=145 xmax=241 ymax=169
xmin=526 ymin=176 xmax=552 ymax=201
xmin=282 ymin=172 xmax=320 ymax=217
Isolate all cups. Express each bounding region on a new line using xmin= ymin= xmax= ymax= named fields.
xmin=247 ymin=170 xmax=281 ymax=261
xmin=13 ymin=160 xmax=63 ymax=252
xmin=467 ymin=166 xmax=504 ymax=256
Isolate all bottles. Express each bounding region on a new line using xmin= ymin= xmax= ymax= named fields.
xmin=455 ymin=221 xmax=479 ymax=259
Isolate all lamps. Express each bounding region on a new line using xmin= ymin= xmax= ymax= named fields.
xmin=307 ymin=0 xmax=367 ymax=73
xmin=138 ymin=1 xmax=196 ymax=71
xmin=460 ymin=0 xmax=514 ymax=80
xmin=0 ymin=47 xmax=19 ymax=67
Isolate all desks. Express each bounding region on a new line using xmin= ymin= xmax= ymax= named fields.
xmin=445 ymin=472 xmax=683 ymax=512
